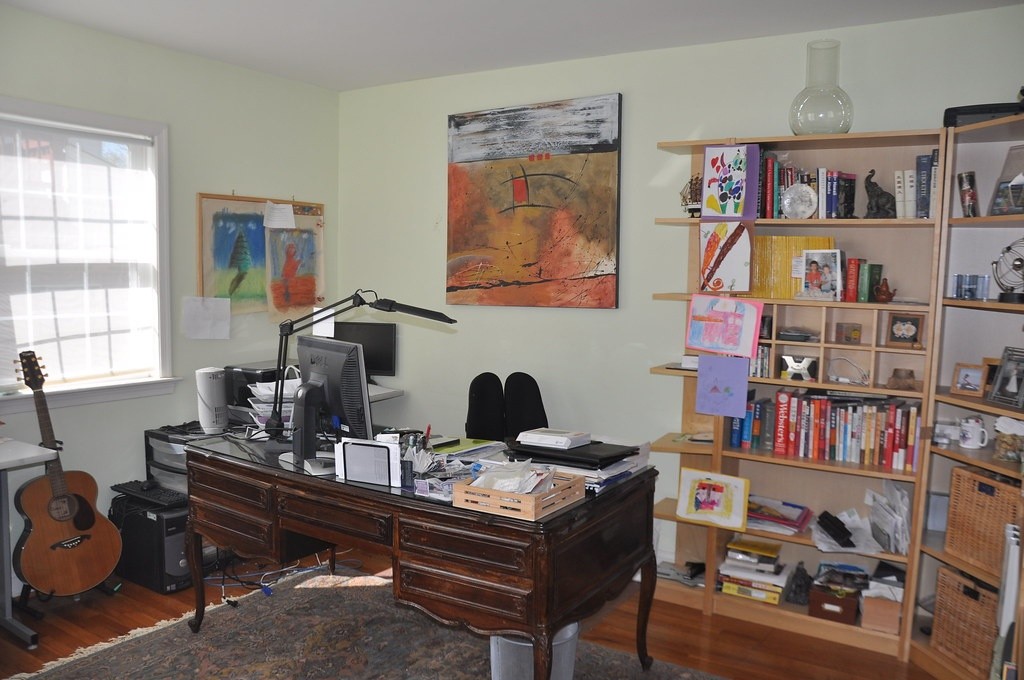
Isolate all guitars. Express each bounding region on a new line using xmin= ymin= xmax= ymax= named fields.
xmin=11 ymin=349 xmax=123 ymax=597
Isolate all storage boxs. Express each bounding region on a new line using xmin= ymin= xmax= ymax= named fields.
xmin=858 ymin=576 xmax=903 ymax=634
xmin=516 ymin=427 xmax=592 ymax=450
xmin=808 ymin=584 xmax=858 ymax=624
xmin=452 ymin=471 xmax=585 ymax=522
xmin=835 ymin=322 xmax=863 ymax=344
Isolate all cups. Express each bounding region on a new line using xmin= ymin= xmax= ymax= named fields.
xmin=958 ymin=418 xmax=989 ymax=450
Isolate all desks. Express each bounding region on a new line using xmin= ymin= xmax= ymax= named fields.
xmin=184 ymin=422 xmax=660 ymax=680
xmin=227 ymin=382 xmax=404 ymax=414
xmin=0 ymin=434 xmax=59 ymax=644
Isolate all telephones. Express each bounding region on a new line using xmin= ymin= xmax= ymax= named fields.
xmin=778 ymin=326 xmax=812 ymax=341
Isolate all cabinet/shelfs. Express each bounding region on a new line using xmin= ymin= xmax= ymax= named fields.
xmin=902 ymin=114 xmax=1024 ymax=680
xmin=645 ymin=126 xmax=947 ymax=661
xmin=143 ymin=419 xmax=258 ymax=574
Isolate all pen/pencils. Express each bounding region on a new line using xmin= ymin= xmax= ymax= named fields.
xmin=665 ymin=367 xmax=697 ymax=371
xmin=686 ymin=437 xmax=713 ymax=443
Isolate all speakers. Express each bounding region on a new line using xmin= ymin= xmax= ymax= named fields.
xmin=195 ymin=366 xmax=229 ymax=434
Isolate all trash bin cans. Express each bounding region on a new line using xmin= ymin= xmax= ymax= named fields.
xmin=489 ymin=621 xmax=579 ymax=680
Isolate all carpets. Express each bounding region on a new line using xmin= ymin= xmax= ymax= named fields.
xmin=7 ymin=557 xmax=735 ymax=680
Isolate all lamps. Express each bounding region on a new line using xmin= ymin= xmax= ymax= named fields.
xmin=264 ymin=288 xmax=457 ymax=438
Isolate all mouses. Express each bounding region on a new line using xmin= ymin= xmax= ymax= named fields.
xmin=140 ymin=480 xmax=156 ymax=491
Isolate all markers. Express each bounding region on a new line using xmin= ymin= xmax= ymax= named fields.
xmin=421 ymin=436 xmax=426 ymax=450
xmin=425 ymin=424 xmax=431 ymax=442
xmin=409 ymin=436 xmax=414 ymax=445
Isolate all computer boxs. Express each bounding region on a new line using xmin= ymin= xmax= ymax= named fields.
xmin=111 ymin=494 xmax=194 ymax=595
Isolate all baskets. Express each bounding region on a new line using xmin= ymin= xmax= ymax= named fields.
xmin=930 ymin=566 xmax=999 ymax=680
xmin=943 ymin=467 xmax=1024 ymax=578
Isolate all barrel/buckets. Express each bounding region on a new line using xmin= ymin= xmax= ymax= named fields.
xmin=490 ymin=620 xmax=580 ymax=680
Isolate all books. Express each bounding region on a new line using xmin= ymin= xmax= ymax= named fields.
xmin=846 ymin=258 xmax=882 ymax=302
xmin=730 ymin=386 xmax=922 ymax=473
xmin=757 ymin=148 xmax=856 ymax=219
xmin=716 ymin=537 xmax=794 ymax=605
xmin=750 ymin=346 xmax=770 ymax=378
xmin=895 ymin=149 xmax=939 ymax=219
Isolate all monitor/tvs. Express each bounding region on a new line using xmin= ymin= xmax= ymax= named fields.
xmin=278 ymin=335 xmax=374 ymax=476
xmin=326 ymin=322 xmax=396 ymax=385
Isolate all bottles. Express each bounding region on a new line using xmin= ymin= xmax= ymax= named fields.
xmin=788 ymin=38 xmax=854 ymax=135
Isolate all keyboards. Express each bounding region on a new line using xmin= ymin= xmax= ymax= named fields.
xmin=110 ymin=479 xmax=189 ymax=506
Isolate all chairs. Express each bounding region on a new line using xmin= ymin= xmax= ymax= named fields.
xmin=465 ymin=371 xmax=548 ymax=442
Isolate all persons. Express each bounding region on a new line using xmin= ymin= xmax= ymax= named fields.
xmin=806 ymin=261 xmax=831 ymax=293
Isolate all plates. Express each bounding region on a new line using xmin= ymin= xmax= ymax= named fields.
xmin=781 ymin=183 xmax=818 ymax=220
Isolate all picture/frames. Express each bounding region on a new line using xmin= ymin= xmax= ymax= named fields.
xmin=986 ymin=345 xmax=1024 ymax=408
xmin=949 ymin=362 xmax=988 ymax=397
xmin=801 ymin=249 xmax=846 ymax=302
xmin=981 ymin=356 xmax=1001 ymax=391
xmin=886 ymin=311 xmax=925 ymax=350
